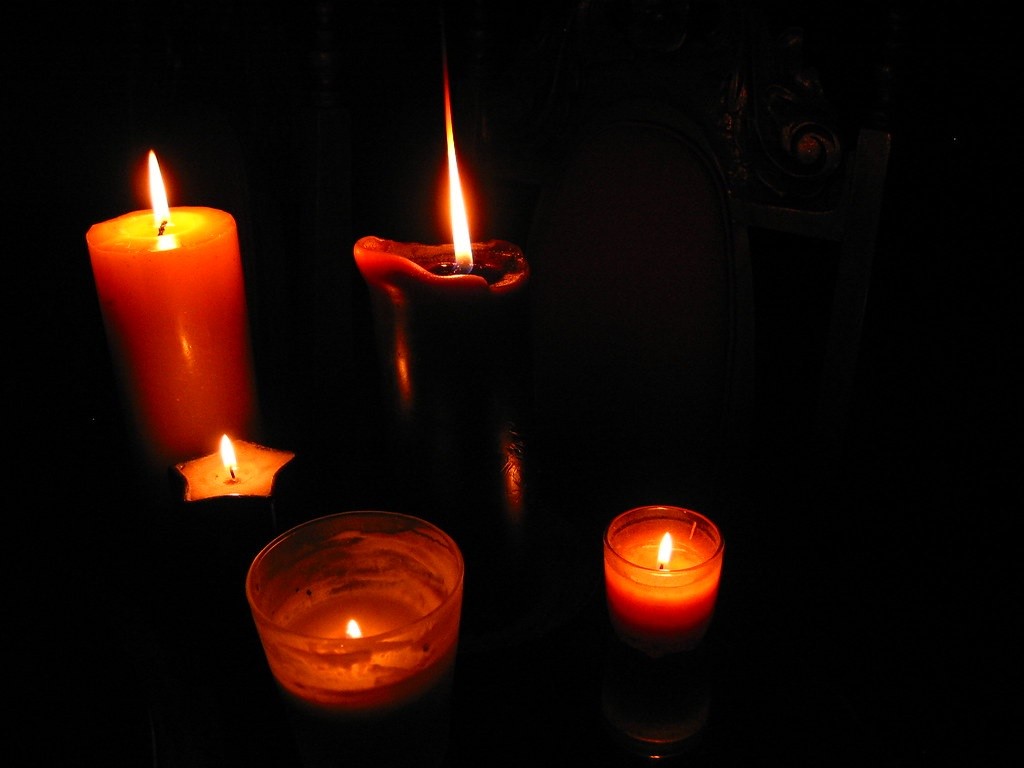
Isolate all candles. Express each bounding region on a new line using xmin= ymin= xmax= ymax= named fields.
xmin=180 ymin=434 xmax=284 ymax=501
xmin=85 ymin=150 xmax=261 ymax=465
xmin=354 ymin=55 xmax=533 ymax=521
xmin=607 ymin=530 xmax=720 ymax=644
xmin=270 ymin=594 xmax=454 ymax=717
